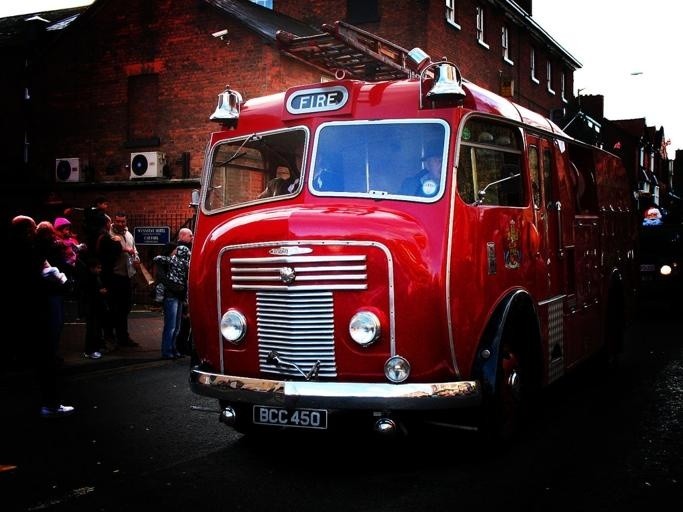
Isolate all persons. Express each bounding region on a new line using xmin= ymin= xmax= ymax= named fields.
xmin=258 ymin=147 xmax=344 ymax=198
xmin=0 ymin=199 xmax=193 ymax=415
xmin=397 ymin=139 xmax=443 ymax=197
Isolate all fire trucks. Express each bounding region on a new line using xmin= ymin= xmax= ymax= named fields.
xmin=187 ymin=45 xmax=633 ymax=451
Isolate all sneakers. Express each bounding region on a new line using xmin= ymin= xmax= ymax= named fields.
xmin=40 ymin=340 xmax=141 ymax=418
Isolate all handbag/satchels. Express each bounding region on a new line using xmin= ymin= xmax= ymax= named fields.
xmin=133 ymin=262 xmax=156 ymax=289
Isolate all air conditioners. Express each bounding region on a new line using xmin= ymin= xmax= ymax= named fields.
xmin=129 ymin=151 xmax=166 ymax=178
xmin=55 ymin=157 xmax=90 ymax=183
